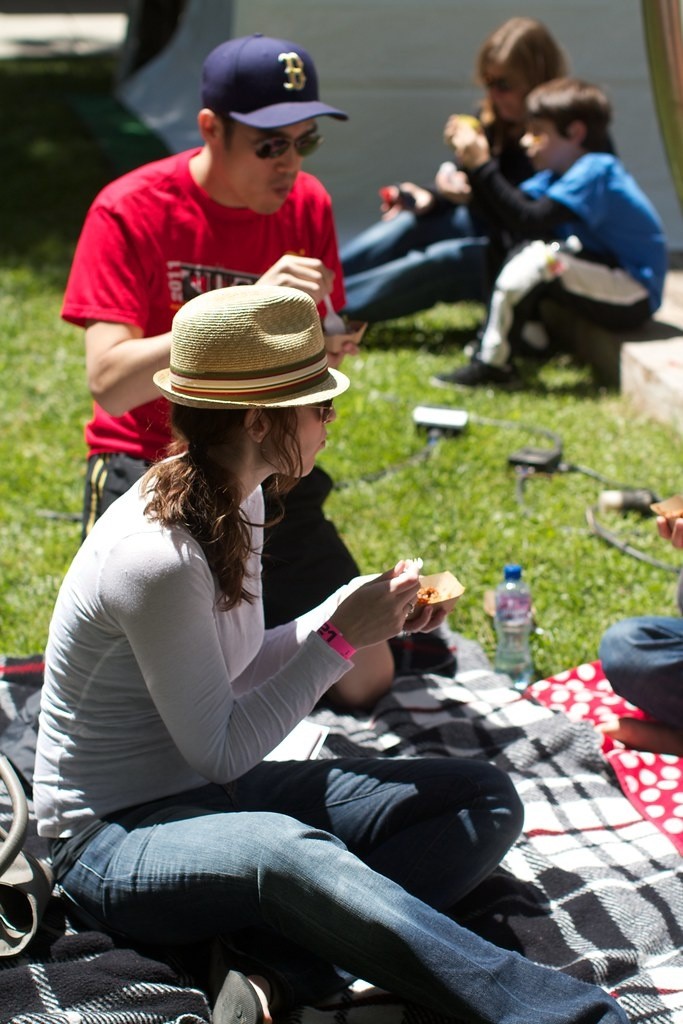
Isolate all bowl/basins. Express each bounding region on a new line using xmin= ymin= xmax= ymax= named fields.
xmin=320 ymin=319 xmax=368 ymax=354
xmin=649 ymin=490 xmax=683 ymax=534
xmin=404 ymin=571 xmax=466 ymax=622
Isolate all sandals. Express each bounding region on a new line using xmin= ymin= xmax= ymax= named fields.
xmin=204 ymin=935 xmax=274 ymax=1024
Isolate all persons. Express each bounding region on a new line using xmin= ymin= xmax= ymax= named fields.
xmin=58 ymin=29 xmax=395 ymax=706
xmin=32 ymin=284 xmax=628 ymax=1023
xmin=338 ymin=16 xmax=666 ymax=386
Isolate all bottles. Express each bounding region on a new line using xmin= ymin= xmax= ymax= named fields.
xmin=492 ymin=562 xmax=537 ymax=693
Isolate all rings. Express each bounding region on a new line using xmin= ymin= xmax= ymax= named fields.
xmin=403 ymin=600 xmax=415 ymax=619
xmin=402 ymin=624 xmax=411 ymax=638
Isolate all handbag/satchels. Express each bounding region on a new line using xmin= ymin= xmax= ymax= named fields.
xmin=0 ymin=756 xmax=51 ymax=959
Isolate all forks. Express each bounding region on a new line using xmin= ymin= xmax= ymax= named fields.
xmin=404 ymin=557 xmax=423 ymax=576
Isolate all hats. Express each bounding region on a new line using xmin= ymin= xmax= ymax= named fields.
xmin=200 ymin=34 xmax=348 ymax=130
xmin=152 ymin=285 xmax=350 ymax=409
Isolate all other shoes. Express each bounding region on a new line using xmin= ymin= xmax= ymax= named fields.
xmin=427 ymin=357 xmax=525 ymax=395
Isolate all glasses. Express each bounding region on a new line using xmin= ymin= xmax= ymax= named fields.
xmin=227 ymin=121 xmax=324 ymax=159
xmin=301 ymin=398 xmax=334 ymax=423
xmin=483 ymin=73 xmax=510 ymax=94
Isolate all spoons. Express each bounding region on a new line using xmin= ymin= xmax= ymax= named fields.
xmin=321 ymin=289 xmax=348 ymax=337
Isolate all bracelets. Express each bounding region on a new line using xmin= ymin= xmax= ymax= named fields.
xmin=317 ymin=619 xmax=357 ymax=661
